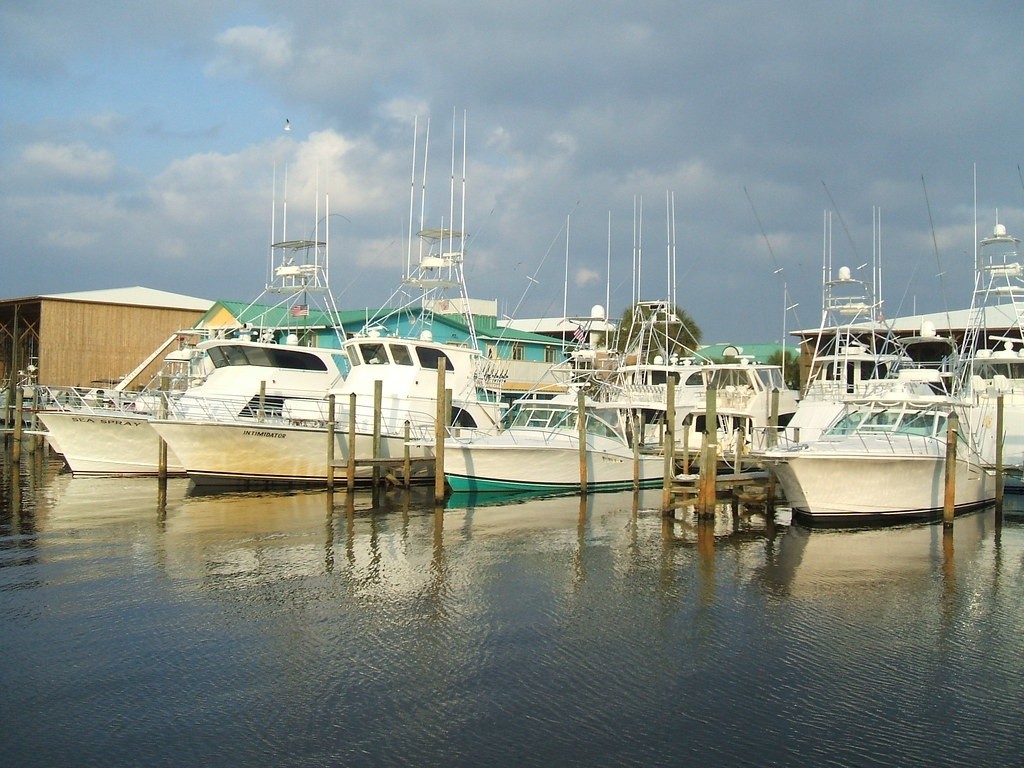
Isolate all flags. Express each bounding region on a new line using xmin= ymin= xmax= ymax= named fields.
xmin=438 ymin=300 xmax=449 ymax=313
xmin=291 ymin=304 xmax=307 ymax=317
xmin=573 ymin=325 xmax=585 ymax=342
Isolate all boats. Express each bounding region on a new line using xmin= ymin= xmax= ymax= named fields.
xmin=27 ymin=100 xmax=1023 ymax=522
